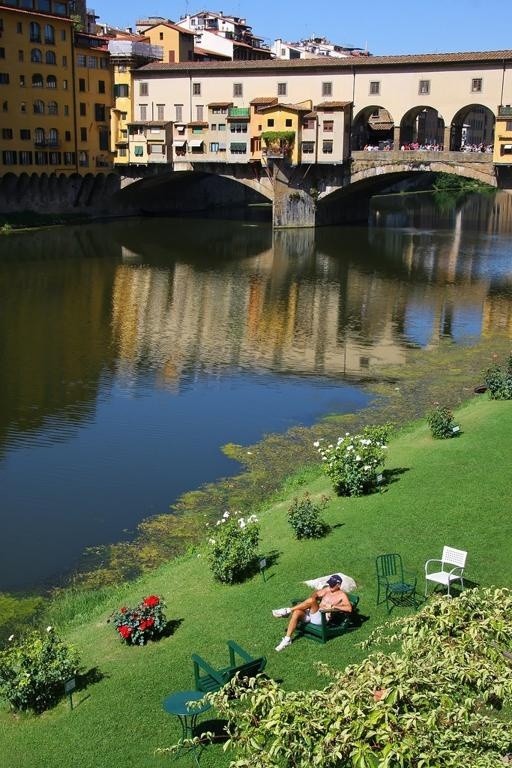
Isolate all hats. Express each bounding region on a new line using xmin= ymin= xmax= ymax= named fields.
xmin=326 ymin=575 xmax=342 ymax=587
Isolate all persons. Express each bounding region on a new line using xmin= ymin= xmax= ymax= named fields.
xmin=272 ymin=575 xmax=353 ymax=652
xmin=364 ymin=142 xmax=494 ymax=153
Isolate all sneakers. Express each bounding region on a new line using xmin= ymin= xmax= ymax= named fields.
xmin=271 ymin=608 xmax=291 ymax=619
xmin=274 ymin=638 xmax=292 ymax=652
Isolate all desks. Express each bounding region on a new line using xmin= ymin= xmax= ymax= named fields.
xmin=162 ymin=691 xmax=218 ymax=768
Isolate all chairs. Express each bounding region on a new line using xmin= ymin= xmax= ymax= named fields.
xmin=424 ymin=545 xmax=468 ymax=597
xmin=375 ymin=553 xmax=418 ymax=614
xmin=287 ymin=592 xmax=360 ymax=644
xmin=190 ymin=641 xmax=267 ymax=703
